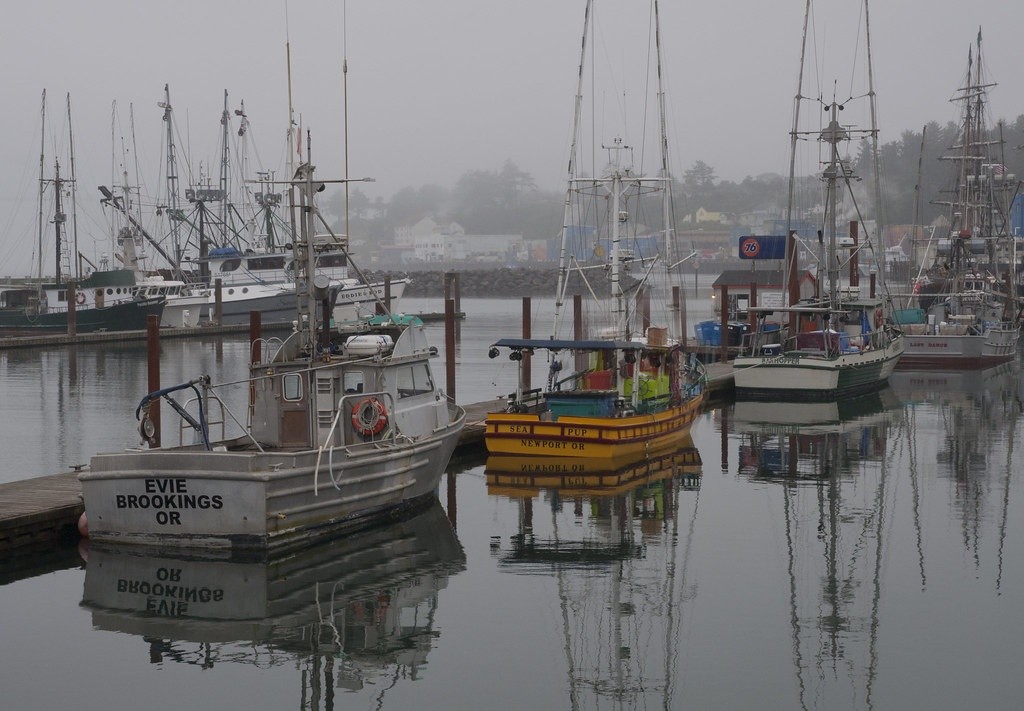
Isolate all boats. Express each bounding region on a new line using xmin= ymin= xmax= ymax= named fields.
xmin=485 ymin=0 xmax=712 ymax=458
xmin=887 ymin=359 xmax=1021 ymax=595
xmin=2 ymin=88 xmax=167 ymax=336
xmin=149 ymin=83 xmax=346 ymax=330
xmin=485 ymin=434 xmax=703 ymax=710
xmin=734 ymin=0 xmax=906 ymax=398
xmin=79 ymin=128 xmax=466 ymax=557
xmin=91 ymin=99 xmax=209 ymax=328
xmin=160 ymin=107 xmax=411 ymax=327
xmin=894 ymin=24 xmax=1022 ymax=374
xmin=80 ymin=501 xmax=466 ymax=711
xmin=734 ymin=382 xmax=907 ymax=710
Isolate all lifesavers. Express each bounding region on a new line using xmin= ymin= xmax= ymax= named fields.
xmin=75 ymin=292 xmax=86 ymax=304
xmin=874 ymin=308 xmax=884 ymax=328
xmin=350 ymin=398 xmax=388 ymax=435
